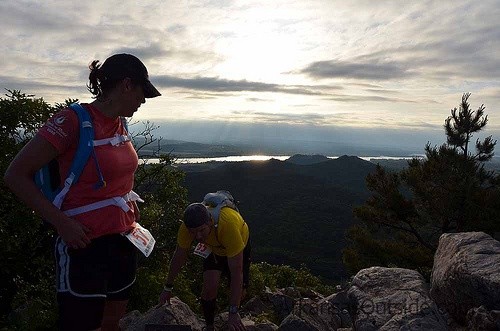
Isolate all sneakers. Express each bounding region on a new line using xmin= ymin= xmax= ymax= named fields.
xmin=247 ymin=280 xmax=254 ymax=298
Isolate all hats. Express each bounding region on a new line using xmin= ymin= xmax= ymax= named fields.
xmin=99 ymin=53 xmax=161 ymax=98
xmin=183 ymin=203 xmax=210 ymax=227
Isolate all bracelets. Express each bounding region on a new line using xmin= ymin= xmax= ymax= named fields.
xmin=229 ymin=306 xmax=239 ymax=314
xmin=164 ymin=284 xmax=173 ymax=292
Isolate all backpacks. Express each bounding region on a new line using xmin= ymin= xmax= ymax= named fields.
xmin=201 ymin=190 xmax=240 ymax=249
xmin=30 ymin=103 xmax=145 ymax=232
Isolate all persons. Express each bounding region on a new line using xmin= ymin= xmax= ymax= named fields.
xmin=159 ymin=203 xmax=250 ymax=331
xmin=4 ymin=53 xmax=161 ymax=331
